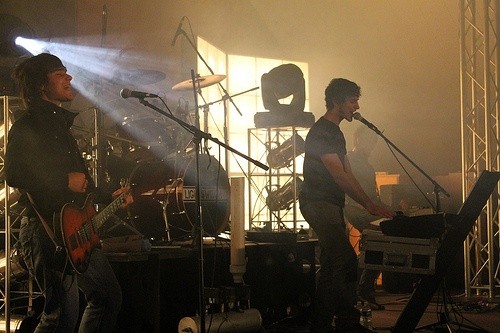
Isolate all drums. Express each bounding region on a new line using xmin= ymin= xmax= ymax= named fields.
xmin=125 ymin=152 xmax=232 ymax=243
xmin=111 ymin=112 xmax=177 ymax=162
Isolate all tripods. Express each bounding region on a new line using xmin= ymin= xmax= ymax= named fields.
xmin=379 ymin=133 xmax=488 ymax=333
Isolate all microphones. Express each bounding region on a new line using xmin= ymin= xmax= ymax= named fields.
xmin=120 ymin=88 xmax=158 ymax=99
xmin=102 ymin=8 xmax=106 ymax=34
xmin=352 ymin=112 xmax=381 ymax=135
xmin=170 ymin=16 xmax=185 ymax=46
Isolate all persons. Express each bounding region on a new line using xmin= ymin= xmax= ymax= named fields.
xmin=343 ymin=125 xmax=409 ymax=310
xmin=299 ymin=78 xmax=397 ymax=333
xmin=4 ymin=53 xmax=134 ymax=333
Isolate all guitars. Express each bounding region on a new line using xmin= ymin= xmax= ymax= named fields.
xmin=51 ymin=179 xmax=134 ymax=278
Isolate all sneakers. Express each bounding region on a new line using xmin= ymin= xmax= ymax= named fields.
xmin=332 ymin=321 xmax=372 ymax=333
xmin=354 ymin=297 xmax=386 ymax=311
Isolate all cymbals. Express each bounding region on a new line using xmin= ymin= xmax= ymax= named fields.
xmin=170 ymin=73 xmax=228 ymax=92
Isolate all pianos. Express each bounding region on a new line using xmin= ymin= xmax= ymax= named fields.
xmin=369 ymin=206 xmax=461 ymax=241
